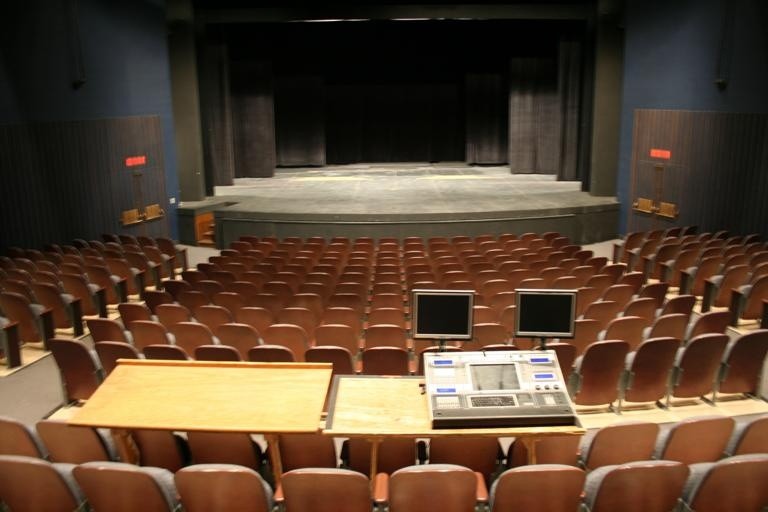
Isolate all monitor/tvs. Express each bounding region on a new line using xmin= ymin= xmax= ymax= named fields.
xmin=513 ymin=288 xmax=579 ymax=339
xmin=411 ymin=289 xmax=475 ymax=340
